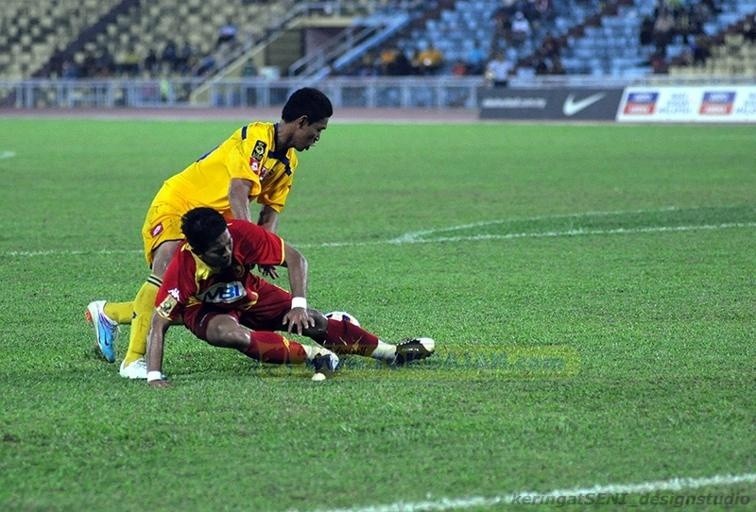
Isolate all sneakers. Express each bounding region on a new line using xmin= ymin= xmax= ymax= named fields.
xmin=120 ymin=358 xmax=167 ymax=379
xmin=85 ymin=300 xmax=118 ymax=363
xmin=311 ymin=348 xmax=340 ymax=382
xmin=388 ymin=337 xmax=434 ymax=367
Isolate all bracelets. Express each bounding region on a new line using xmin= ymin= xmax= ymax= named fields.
xmin=146 ymin=371 xmax=161 ymax=382
xmin=290 ymin=297 xmax=308 ymax=310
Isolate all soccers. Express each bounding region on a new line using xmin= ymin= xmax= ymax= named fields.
xmin=324 ymin=311 xmax=361 ymax=327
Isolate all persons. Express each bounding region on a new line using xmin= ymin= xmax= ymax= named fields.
xmin=144 ymin=205 xmax=439 ymax=383
xmin=85 ymin=86 xmax=334 ymax=381
